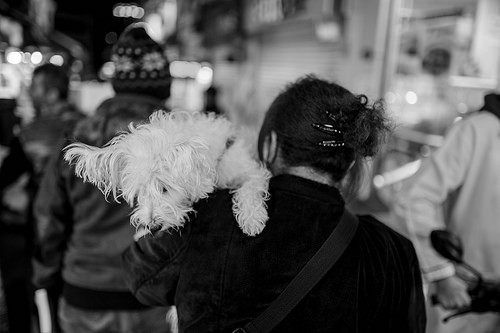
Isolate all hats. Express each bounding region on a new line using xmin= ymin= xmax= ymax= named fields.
xmin=110 ymin=27 xmax=172 ymax=98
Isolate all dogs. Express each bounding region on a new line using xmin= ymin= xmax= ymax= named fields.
xmin=62 ymin=106 xmax=271 ymax=240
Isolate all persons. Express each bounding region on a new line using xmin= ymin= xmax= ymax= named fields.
xmin=404 ymin=91 xmax=500 ymax=333
xmin=0 ymin=23 xmax=174 ymax=333
xmin=118 ymin=77 xmax=429 ymax=333
